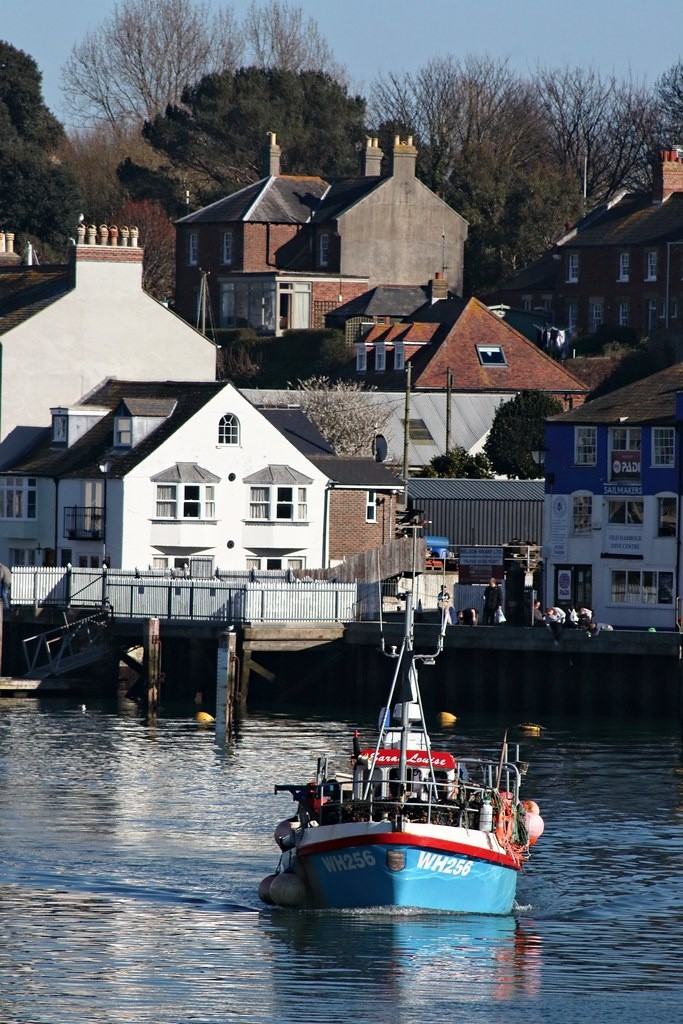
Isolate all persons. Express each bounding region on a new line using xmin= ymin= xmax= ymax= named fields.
xmin=534 ymin=601 xmax=613 ymax=637
xmin=438 ymin=583 xmax=479 ymax=625
xmin=483 ymin=578 xmax=503 ymax=625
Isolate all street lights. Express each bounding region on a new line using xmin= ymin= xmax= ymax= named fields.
xmin=412 ymin=520 xmax=432 ymax=609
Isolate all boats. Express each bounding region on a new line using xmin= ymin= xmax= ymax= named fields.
xmin=279 ymin=591 xmax=523 ymax=917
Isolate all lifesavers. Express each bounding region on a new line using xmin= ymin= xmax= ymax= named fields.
xmin=497 ymin=805 xmax=514 ymax=847
